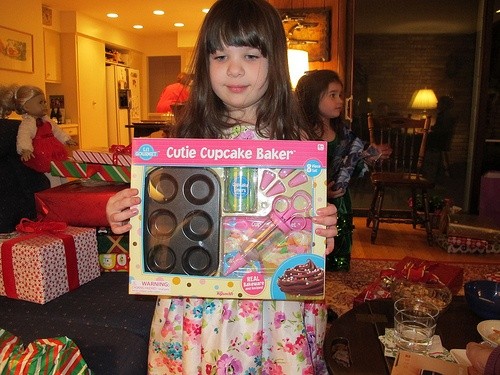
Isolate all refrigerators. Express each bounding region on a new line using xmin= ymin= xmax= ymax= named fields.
xmin=106 ymin=64 xmax=140 ymax=148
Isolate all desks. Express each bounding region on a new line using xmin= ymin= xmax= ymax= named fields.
xmin=323 ymin=295 xmax=500 ymax=375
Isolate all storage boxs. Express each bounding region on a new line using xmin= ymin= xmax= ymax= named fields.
xmin=50 ymin=160 xmax=131 ymax=183
xmin=127 ymin=137 xmax=327 ymax=301
xmin=0 ymin=225 xmax=100 ymax=306
xmin=34 ymin=178 xmax=131 ymax=227
xmin=353 ymin=255 xmax=465 ymax=316
xmin=72 ymin=146 xmax=132 ymax=166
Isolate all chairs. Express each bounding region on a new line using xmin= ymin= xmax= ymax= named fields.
xmin=366 ymin=111 xmax=434 ymax=246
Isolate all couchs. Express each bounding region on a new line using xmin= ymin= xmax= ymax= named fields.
xmin=0 ymin=116 xmax=157 ymax=375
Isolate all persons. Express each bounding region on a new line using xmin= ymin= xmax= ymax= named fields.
xmin=107 ymin=0 xmax=338 ymax=375
xmin=466 ymin=342 xmax=500 ymax=375
xmin=295 ymin=68 xmax=352 ymax=272
xmin=156 ymin=72 xmax=192 ymax=113
xmin=0 ymin=83 xmax=78 ymax=172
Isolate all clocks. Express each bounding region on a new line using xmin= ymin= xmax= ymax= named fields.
xmin=41 ymin=6 xmax=53 ymax=25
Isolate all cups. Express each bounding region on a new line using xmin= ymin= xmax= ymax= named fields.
xmin=394 ymin=309 xmax=437 ymax=357
xmin=393 ymin=298 xmax=439 ymax=327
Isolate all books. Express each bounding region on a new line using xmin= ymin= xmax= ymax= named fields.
xmin=132 ymin=113 xmax=176 ymax=124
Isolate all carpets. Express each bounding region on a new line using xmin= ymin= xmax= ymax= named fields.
xmin=325 ymin=257 xmax=500 ymax=327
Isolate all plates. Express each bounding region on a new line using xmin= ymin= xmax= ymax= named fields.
xmin=450 ymin=349 xmax=473 ymax=368
xmin=383 ymin=328 xmax=443 ymax=357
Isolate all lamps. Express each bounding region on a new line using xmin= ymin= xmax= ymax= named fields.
xmin=411 ymin=89 xmax=438 ymax=121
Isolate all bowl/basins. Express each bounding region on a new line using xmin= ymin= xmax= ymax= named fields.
xmin=477 ymin=319 xmax=500 ymax=349
xmin=464 ymin=279 xmax=500 ymax=320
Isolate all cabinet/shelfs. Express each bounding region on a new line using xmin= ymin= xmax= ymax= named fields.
xmin=47 ymin=33 xmax=140 ymax=153
xmin=42 ymin=28 xmax=62 ymax=84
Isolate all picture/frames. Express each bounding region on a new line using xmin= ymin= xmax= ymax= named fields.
xmin=277 ymin=5 xmax=331 ymax=62
xmin=0 ymin=25 xmax=35 ymax=73
